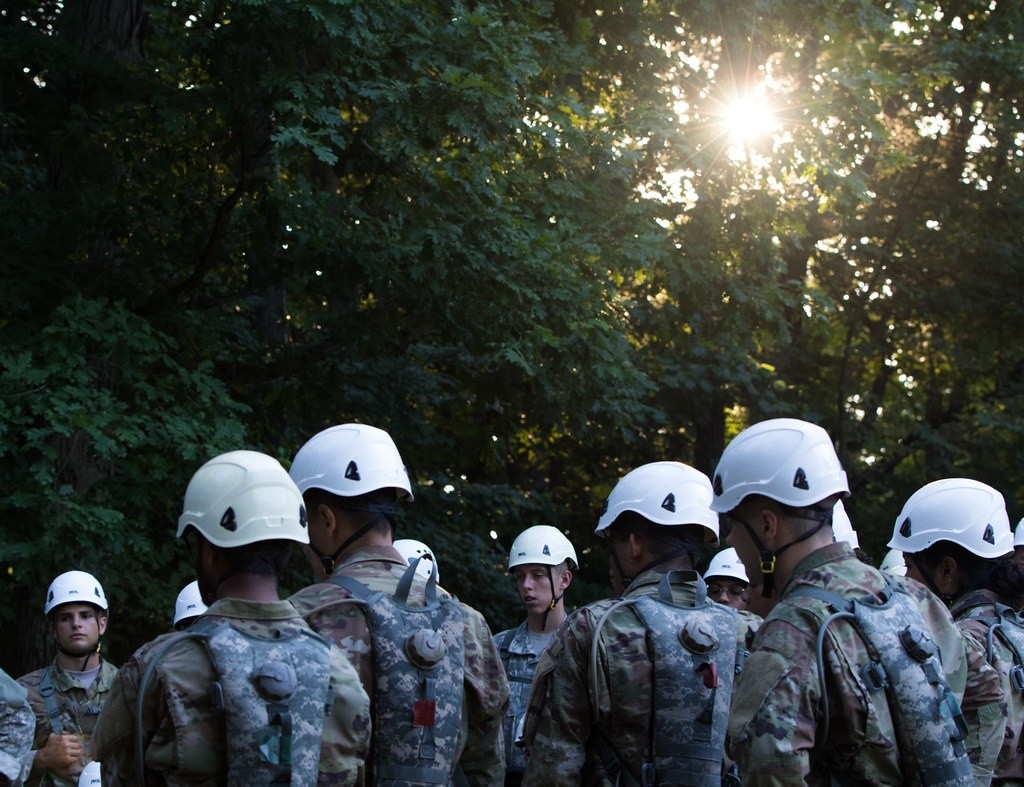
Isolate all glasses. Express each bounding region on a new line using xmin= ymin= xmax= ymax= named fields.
xmin=708 ymin=582 xmax=748 ymax=595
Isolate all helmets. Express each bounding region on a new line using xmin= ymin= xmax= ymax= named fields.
xmin=879 ymin=549 xmax=908 ymax=577
xmin=173 ymin=579 xmax=209 ymax=628
xmin=177 ymin=450 xmax=310 ymax=547
xmin=703 ymin=548 xmax=750 ymax=583
xmin=393 ymin=540 xmax=440 ymax=584
xmin=289 ymin=423 xmax=414 ymax=502
xmin=509 ymin=525 xmax=579 ymax=570
xmin=886 ymin=478 xmax=1015 ymax=559
xmin=710 ymin=418 xmax=851 ymax=513
xmin=43 ymin=571 xmax=107 ymax=614
xmin=1013 ymin=518 xmax=1024 ymax=547
xmin=832 ymin=499 xmax=860 ymax=549
xmin=594 ymin=461 xmax=720 ymax=548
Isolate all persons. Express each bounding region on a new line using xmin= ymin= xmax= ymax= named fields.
xmin=16 ymin=570 xmax=120 ymax=787
xmin=493 ymin=525 xmax=580 ymax=787
xmin=516 ymin=418 xmax=1024 ymax=787
xmin=282 ymin=423 xmax=510 ymax=787
xmin=0 ymin=667 xmax=36 ymax=787
xmin=91 ymin=450 xmax=372 ymax=787
